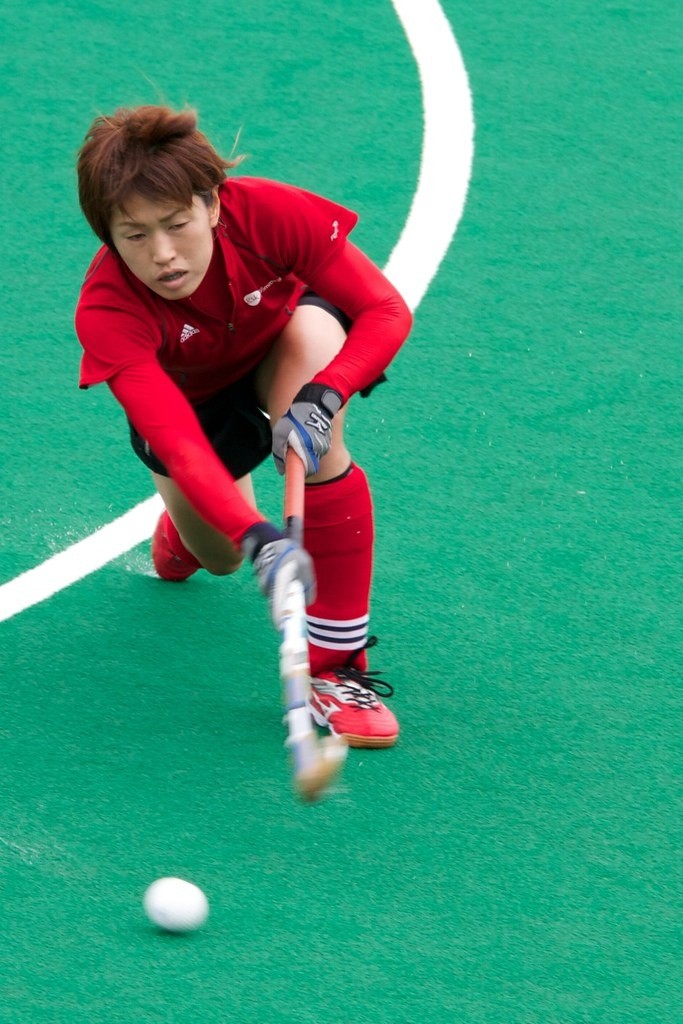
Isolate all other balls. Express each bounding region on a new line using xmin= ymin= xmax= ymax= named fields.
xmin=141 ymin=874 xmax=212 ymax=935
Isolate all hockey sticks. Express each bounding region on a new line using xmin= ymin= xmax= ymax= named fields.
xmin=272 ymin=441 xmax=354 ymax=801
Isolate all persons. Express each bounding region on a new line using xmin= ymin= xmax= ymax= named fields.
xmin=76 ymin=107 xmax=414 ymax=749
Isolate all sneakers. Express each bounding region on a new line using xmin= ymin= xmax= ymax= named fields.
xmin=152 ymin=509 xmax=196 ymax=582
xmin=307 ymin=636 xmax=398 ymax=748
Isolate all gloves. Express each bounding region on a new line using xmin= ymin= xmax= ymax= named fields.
xmin=273 ymin=382 xmax=343 ymax=476
xmin=240 ymin=519 xmax=317 ymax=608
